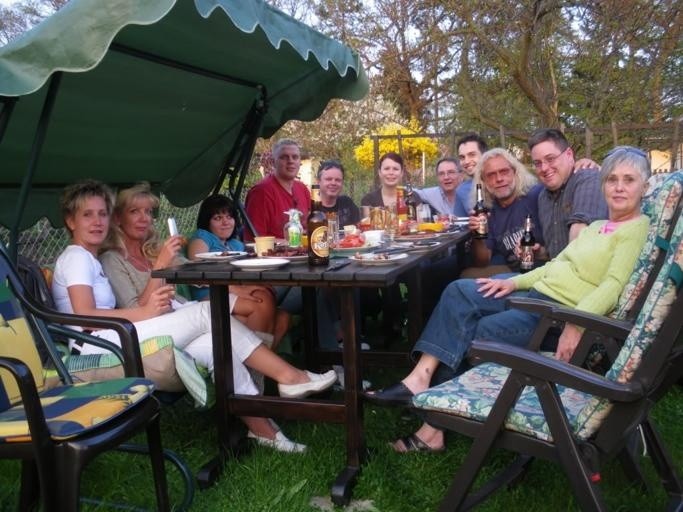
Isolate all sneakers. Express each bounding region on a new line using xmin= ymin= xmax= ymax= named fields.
xmin=325 ymin=336 xmax=372 ymax=393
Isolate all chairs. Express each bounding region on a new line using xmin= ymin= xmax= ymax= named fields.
xmin=2 ymin=170 xmax=680 ymax=512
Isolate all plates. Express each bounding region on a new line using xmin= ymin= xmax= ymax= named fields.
xmin=227 ymin=256 xmax=290 ymax=272
xmin=258 ymin=253 xmax=308 ymax=264
xmin=244 ymin=240 xmax=288 ymax=250
xmin=329 ymin=216 xmax=469 ymax=251
xmin=193 ymin=251 xmax=247 ymax=262
xmin=350 ymin=254 xmax=407 ymax=265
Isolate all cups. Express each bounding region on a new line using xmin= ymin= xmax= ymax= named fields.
xmin=368 ymin=206 xmax=393 ymax=235
xmin=253 ymin=236 xmax=274 ymax=256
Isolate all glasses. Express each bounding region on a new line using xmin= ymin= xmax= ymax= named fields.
xmin=532 ymin=149 xmax=567 ymax=166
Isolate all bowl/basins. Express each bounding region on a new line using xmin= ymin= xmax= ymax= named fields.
xmin=300 ymin=235 xmax=307 ymax=249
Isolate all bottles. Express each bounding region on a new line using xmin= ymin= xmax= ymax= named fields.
xmin=518 ymin=214 xmax=534 ymax=273
xmin=472 ymin=182 xmax=488 ymax=240
xmin=403 ymin=183 xmax=416 ymax=226
xmin=394 ymin=186 xmax=408 ymax=236
xmin=306 ymin=184 xmax=330 ymax=266
xmin=282 ymin=209 xmax=303 ymax=247
xmin=421 ymin=204 xmax=431 ymax=223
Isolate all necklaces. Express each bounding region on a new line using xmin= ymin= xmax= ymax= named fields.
xmin=129 ymin=255 xmax=151 ymax=272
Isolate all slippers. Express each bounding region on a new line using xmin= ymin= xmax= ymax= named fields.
xmin=386 ymin=434 xmax=448 ymax=455
xmin=366 ymin=382 xmax=418 ymax=407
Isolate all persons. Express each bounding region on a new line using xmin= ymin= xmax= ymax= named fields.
xmin=358 ymin=144 xmax=650 ymax=454
xmin=50 ymin=178 xmax=337 ymax=454
xmin=100 ymin=181 xmax=291 ymax=353
xmin=185 ymin=194 xmax=247 ymax=300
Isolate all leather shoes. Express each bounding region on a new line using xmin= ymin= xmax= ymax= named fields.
xmin=248 ymin=427 xmax=307 ymax=454
xmin=277 ymin=371 xmax=338 ymax=398
